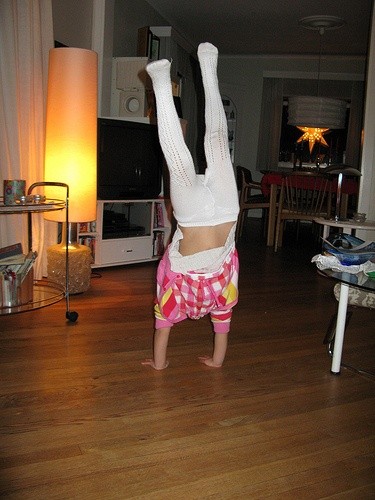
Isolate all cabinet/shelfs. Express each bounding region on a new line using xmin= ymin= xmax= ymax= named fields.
xmin=0 ymin=182 xmax=78 ymax=323
xmin=77 ymin=200 xmax=164 ymax=269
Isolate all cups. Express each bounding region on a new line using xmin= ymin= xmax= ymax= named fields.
xmin=4 ymin=180 xmax=26 ymax=205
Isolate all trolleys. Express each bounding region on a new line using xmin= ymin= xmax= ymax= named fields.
xmin=0 ymin=179 xmax=80 ymax=322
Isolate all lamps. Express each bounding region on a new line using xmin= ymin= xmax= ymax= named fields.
xmin=44 ymin=47 xmax=97 ymax=295
xmin=286 ymin=15 xmax=346 ymax=129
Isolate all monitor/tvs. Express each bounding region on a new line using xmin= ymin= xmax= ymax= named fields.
xmin=97 ymin=118 xmax=164 ymax=200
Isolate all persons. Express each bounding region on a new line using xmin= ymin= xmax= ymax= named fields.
xmin=143 ymin=41 xmax=242 ymax=372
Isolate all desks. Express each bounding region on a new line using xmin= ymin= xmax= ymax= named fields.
xmin=259 ymin=167 xmax=358 ymax=246
xmin=315 ymin=247 xmax=375 ymax=375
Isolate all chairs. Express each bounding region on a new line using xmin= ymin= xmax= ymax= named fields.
xmin=324 ymin=282 xmax=375 ymax=381
xmin=236 ymin=166 xmax=284 ymax=248
xmin=274 ymin=172 xmax=333 ymax=253
